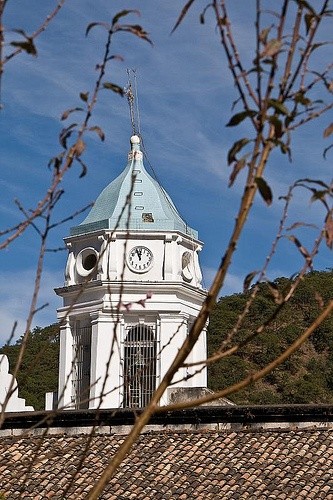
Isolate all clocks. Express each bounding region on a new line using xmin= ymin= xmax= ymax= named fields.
xmin=127 ymin=246 xmax=154 ymax=274
